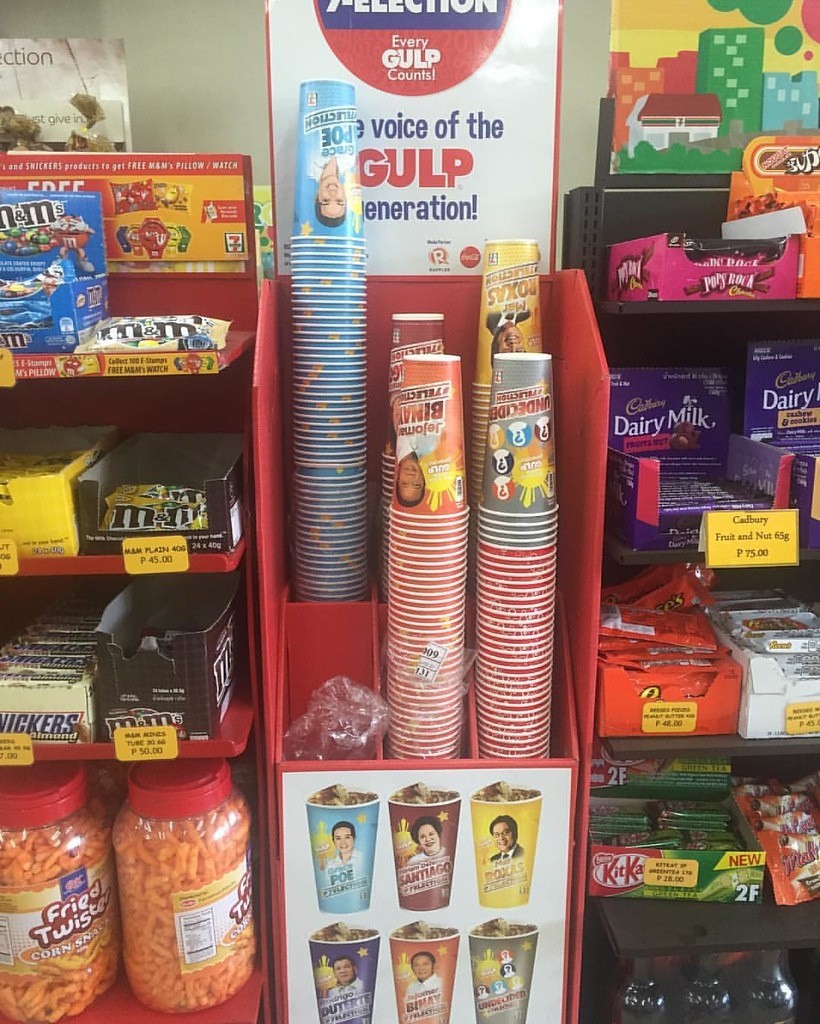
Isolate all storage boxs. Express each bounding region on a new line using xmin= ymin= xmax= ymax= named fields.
xmin=0 ymin=189 xmax=109 ymax=354
xmin=0 ymin=424 xmax=121 ymax=557
xmin=606 ymin=232 xmax=799 ymax=302
xmin=76 ymin=431 xmax=243 ymax=553
xmin=605 ymin=367 xmax=796 ymax=551
xmin=589 ymin=747 xmax=768 ymax=905
xmin=740 ymin=340 xmax=820 ymax=548
xmin=724 ymin=135 xmax=819 ymax=300
xmin=699 ymin=590 xmax=820 ymax=739
xmin=596 ymin=651 xmax=743 ymax=740
xmin=93 ymin=572 xmax=241 ymax=739
xmin=0 ymin=600 xmax=97 ymax=744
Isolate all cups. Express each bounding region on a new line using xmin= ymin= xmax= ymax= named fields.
xmin=306 ymin=788 xmax=543 ymax=1024
xmin=294 ymin=80 xmax=552 ymax=763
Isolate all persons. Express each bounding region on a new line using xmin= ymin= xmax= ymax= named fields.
xmin=327 ymin=955 xmax=364 ymax=1000
xmin=407 ymin=815 xmax=447 ymax=864
xmin=485 ymin=307 xmax=531 ymax=368
xmin=489 ymin=814 xmax=524 ymax=863
xmin=327 ymin=820 xmax=366 ymax=883
xmin=395 ymin=417 xmax=444 ymax=508
xmin=307 ymin=152 xmax=355 ymax=228
xmin=404 ymin=951 xmax=442 ymax=1005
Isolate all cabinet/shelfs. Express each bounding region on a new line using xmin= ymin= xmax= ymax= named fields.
xmin=252 ymin=269 xmax=612 ymax=1023
xmin=560 ymin=181 xmax=820 ymax=1024
xmin=0 ymin=151 xmax=271 ymax=1024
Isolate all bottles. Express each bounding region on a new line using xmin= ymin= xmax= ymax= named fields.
xmin=1 ymin=752 xmax=256 ymax=1023
